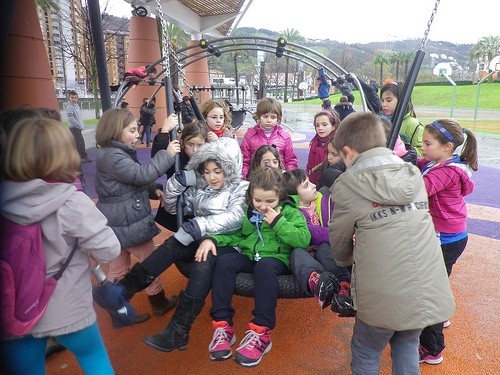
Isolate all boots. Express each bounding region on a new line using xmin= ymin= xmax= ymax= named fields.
xmin=90 ymin=262 xmax=154 ymax=312
xmin=142 ymin=289 xmax=206 ymax=353
xmin=146 ymin=290 xmax=178 ymax=318
xmin=132 ymin=310 xmax=151 ymax=324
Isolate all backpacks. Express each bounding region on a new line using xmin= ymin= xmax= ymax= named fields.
xmin=0 ymin=211 xmax=79 ymax=340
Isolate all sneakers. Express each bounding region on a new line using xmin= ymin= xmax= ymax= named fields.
xmin=310 ymin=271 xmax=340 ymax=310
xmin=331 ymin=292 xmax=357 ymax=319
xmin=442 ymin=319 xmax=452 ymax=329
xmin=208 ymin=320 xmax=237 ymax=362
xmin=417 ymin=344 xmax=444 ymax=365
xmin=234 ymin=322 xmax=274 ymax=368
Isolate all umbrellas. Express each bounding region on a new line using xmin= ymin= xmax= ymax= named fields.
xmin=90 ymin=265 xmax=137 ymax=325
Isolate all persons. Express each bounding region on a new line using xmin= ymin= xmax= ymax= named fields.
xmin=380 ymin=79 xmax=425 ymax=158
xmin=95 ymin=109 xmax=182 ymax=325
xmin=416 ymin=118 xmax=478 ymax=364
xmin=149 ymin=112 xmax=219 ymax=233
xmin=283 ymin=168 xmax=356 ymax=318
xmin=240 ymin=99 xmax=298 ymax=178
xmin=180 ymin=96 xmax=197 ymax=124
xmin=353 ymin=77 xmax=381 ymax=113
xmin=118 ymin=138 xmax=250 ymax=352
xmin=195 ymin=165 xmax=311 ymax=368
xmin=193 ymin=100 xmax=243 ymax=176
xmin=306 ymin=109 xmax=340 ymax=188
xmin=253 ymin=145 xmax=283 ymax=172
xmin=0 ymin=117 xmax=122 ymax=375
xmin=63 ymin=91 xmax=91 ymax=162
xmin=330 ymin=114 xmax=455 ymax=375
xmin=122 ymin=96 xmax=158 ymax=144
xmin=317 ymin=67 xmax=355 ymax=119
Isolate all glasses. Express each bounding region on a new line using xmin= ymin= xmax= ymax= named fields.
xmin=207 ymin=115 xmax=223 ymax=120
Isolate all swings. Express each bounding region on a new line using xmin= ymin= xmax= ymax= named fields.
xmin=156 ymin=0 xmax=440 ymax=299
